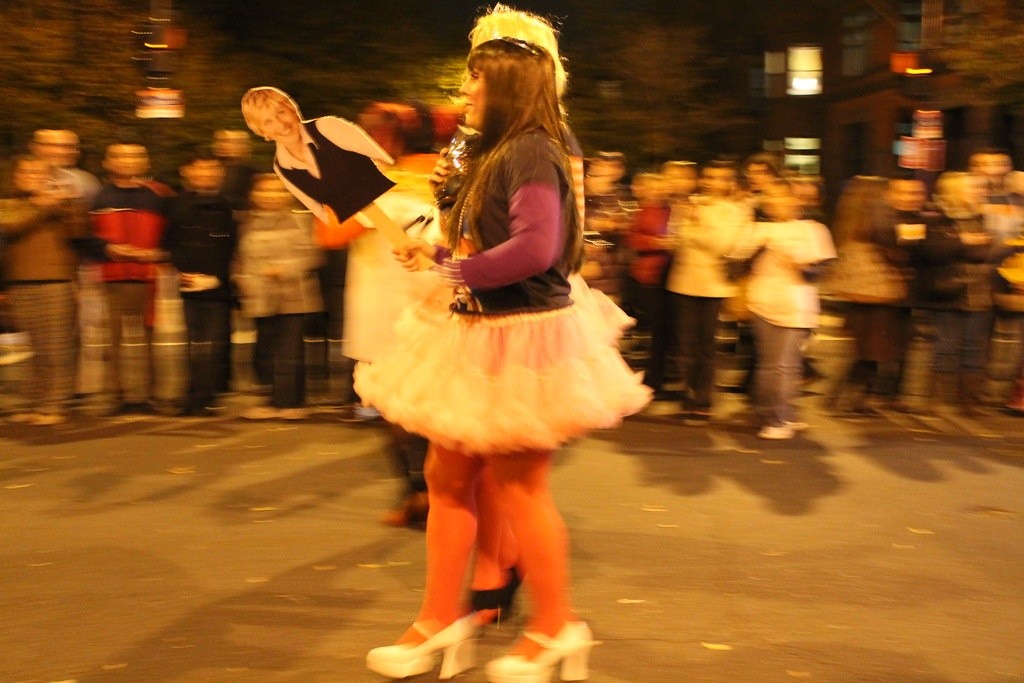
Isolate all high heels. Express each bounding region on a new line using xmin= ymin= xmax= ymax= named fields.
xmin=365 ymin=610 xmax=478 ymax=681
xmin=484 ymin=618 xmax=593 ymax=683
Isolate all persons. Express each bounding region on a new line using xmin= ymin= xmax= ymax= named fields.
xmin=309 ymin=5 xmax=653 ymax=683
xmin=2 ymin=102 xmax=1024 ymax=439
xmin=241 ymin=87 xmax=399 ymax=228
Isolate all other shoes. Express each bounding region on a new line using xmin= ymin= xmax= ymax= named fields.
xmin=381 ymin=490 xmax=430 ymax=529
xmin=469 ymin=568 xmax=519 ymax=623
xmin=9 ymin=384 xmax=941 ymax=441
